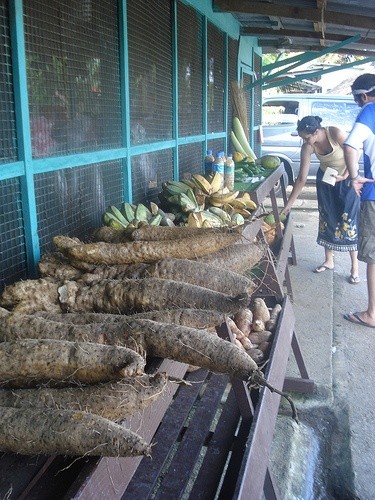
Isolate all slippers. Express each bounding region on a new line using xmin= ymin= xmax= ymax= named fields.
xmin=312 ymin=265 xmax=334 ymax=272
xmin=344 ymin=312 xmax=375 ymax=327
xmin=350 ymin=274 xmax=360 ymax=283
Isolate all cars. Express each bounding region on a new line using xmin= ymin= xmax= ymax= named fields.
xmin=258 ymin=94 xmax=365 ymax=183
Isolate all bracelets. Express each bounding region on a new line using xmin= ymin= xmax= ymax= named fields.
xmin=347 ymin=174 xmax=359 ymax=181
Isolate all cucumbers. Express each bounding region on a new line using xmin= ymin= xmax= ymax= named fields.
xmin=234 ymin=157 xmax=265 ymax=178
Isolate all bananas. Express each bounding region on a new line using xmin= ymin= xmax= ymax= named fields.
xmin=98 ymin=172 xmax=257 ymax=229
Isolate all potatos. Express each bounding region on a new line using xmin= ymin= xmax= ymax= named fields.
xmin=206 ymin=298 xmax=282 ymax=361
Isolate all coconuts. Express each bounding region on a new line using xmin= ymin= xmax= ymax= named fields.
xmin=264 ymin=210 xmax=287 ymax=226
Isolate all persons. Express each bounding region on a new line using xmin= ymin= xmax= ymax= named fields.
xmin=28 ymin=92 xmax=158 ymax=230
xmin=278 ymin=115 xmax=362 ymax=284
xmin=343 ymin=72 xmax=375 ymax=329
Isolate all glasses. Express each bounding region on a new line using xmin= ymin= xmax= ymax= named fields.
xmin=297 ymin=119 xmax=316 ymax=129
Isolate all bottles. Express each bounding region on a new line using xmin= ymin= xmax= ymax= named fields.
xmin=205 ymin=150 xmax=215 ymax=177
xmin=214 ymin=153 xmax=224 ymax=178
xmin=220 ymin=151 xmax=226 ymax=162
xmin=224 ymin=154 xmax=235 ymax=191
